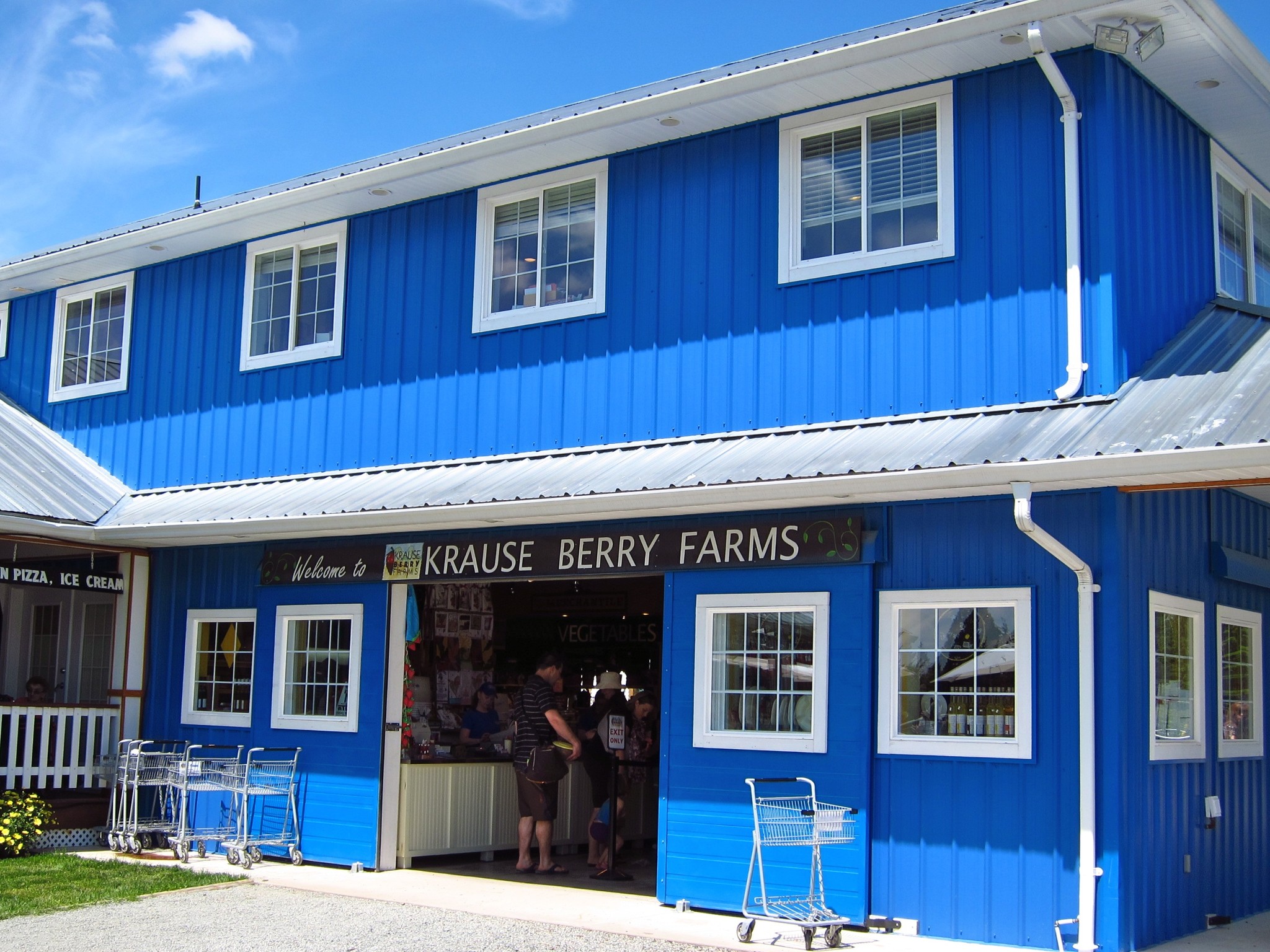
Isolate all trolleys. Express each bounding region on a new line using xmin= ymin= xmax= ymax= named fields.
xmin=91 ymin=739 xmax=192 ymax=855
xmin=735 ymin=775 xmax=860 ymax=950
xmin=167 ymin=744 xmax=304 ymax=869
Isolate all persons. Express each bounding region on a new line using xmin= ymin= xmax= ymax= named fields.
xmin=512 ymin=650 xmax=581 ymax=875
xmin=12 ymin=676 xmax=54 ymax=726
xmin=553 ymin=670 xmax=657 ymax=867
xmin=458 ymin=682 xmax=501 ymax=759
xmin=1223 ymin=703 xmax=1250 ymax=739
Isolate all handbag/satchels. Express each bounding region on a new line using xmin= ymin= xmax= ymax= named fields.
xmin=524 ymin=746 xmax=569 ymax=785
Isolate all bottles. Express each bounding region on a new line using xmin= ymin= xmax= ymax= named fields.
xmin=948 ymin=686 xmax=1014 ymax=737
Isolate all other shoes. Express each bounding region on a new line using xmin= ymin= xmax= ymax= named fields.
xmin=596 ymin=862 xmax=608 ymax=870
xmin=587 ymin=856 xmax=598 ymax=867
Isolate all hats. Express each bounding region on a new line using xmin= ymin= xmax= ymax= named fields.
xmin=481 ymin=681 xmax=498 ymax=699
xmin=593 ymin=671 xmax=627 ymax=689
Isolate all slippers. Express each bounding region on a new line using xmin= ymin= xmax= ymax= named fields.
xmin=514 ymin=863 xmax=538 ymax=873
xmin=535 ymin=864 xmax=570 ymax=874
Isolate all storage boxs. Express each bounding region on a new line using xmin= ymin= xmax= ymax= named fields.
xmin=524 ymin=290 xmax=562 ymax=307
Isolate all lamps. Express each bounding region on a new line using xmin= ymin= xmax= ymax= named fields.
xmin=1094 ymin=18 xmax=1165 ymax=64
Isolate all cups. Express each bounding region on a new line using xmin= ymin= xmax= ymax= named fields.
xmin=504 ymin=740 xmax=512 ymax=753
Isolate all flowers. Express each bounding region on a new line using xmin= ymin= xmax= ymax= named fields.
xmin=401 ymin=628 xmax=421 ymax=747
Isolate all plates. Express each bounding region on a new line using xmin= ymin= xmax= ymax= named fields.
xmin=737 ymin=687 xmax=812 ymax=731
xmin=921 ymin=691 xmax=947 ymax=721
